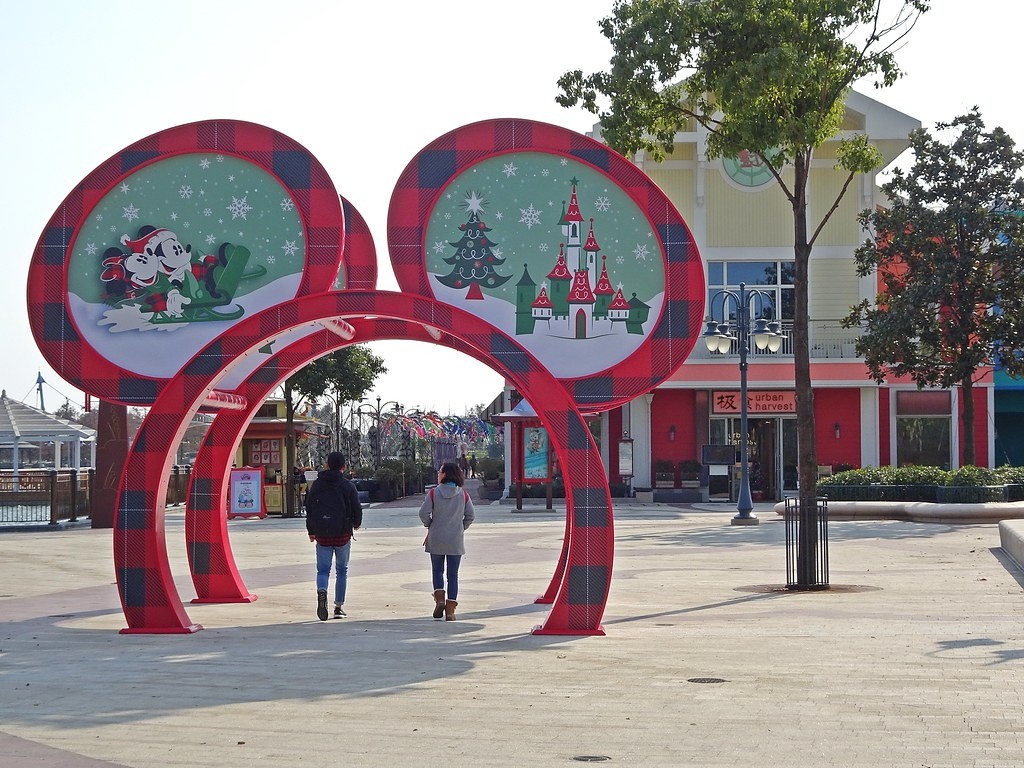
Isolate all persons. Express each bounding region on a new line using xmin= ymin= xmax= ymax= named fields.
xmin=419 ymin=462 xmax=475 ymax=621
xmin=457 ymin=453 xmax=477 ymax=480
xmin=304 ymin=450 xmax=362 ymax=621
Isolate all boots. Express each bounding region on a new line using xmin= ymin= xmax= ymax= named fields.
xmin=431 ymin=589 xmax=446 ymax=618
xmin=445 ymin=599 xmax=459 ymax=621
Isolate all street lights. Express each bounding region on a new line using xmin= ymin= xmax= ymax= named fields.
xmin=322 ymin=390 xmax=363 ymax=452
xmin=702 ymin=281 xmax=787 ymax=527
xmin=356 ymin=395 xmax=399 ymax=472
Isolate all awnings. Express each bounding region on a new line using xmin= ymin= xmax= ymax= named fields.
xmin=480 ymin=391 xmax=505 ymax=424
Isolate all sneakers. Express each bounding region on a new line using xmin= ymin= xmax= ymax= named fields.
xmin=334 ymin=609 xmax=347 ymax=618
xmin=317 ymin=590 xmax=328 ymax=621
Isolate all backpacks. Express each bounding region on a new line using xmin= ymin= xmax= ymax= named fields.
xmin=311 ymin=479 xmax=351 ymax=536
